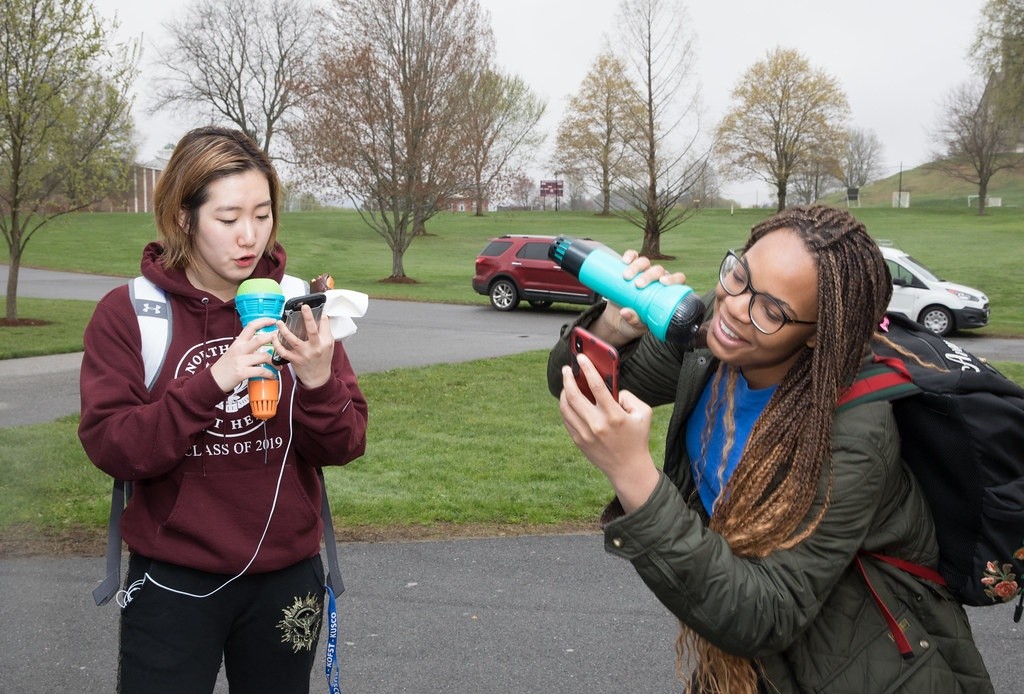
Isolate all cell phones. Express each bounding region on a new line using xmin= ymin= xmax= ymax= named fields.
xmin=272 ymin=293 xmax=326 ymax=366
xmin=570 ymin=326 xmax=619 ymax=407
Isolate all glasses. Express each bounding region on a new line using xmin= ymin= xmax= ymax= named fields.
xmin=718 ymin=246 xmax=818 ymax=336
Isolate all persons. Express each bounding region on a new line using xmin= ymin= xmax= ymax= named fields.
xmin=76 ymin=126 xmax=367 ymax=693
xmin=545 ymin=202 xmax=997 ymax=693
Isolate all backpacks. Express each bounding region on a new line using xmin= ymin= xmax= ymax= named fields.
xmin=832 ymin=309 xmax=1024 ymax=656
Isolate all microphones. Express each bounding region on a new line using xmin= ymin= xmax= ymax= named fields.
xmin=547 ymin=234 xmax=706 ymax=345
xmin=235 ymin=278 xmax=286 ymax=464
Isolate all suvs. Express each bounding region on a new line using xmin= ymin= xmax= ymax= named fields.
xmin=472 ymin=233 xmax=605 ymax=312
xmin=877 ymin=246 xmax=990 ymax=338
